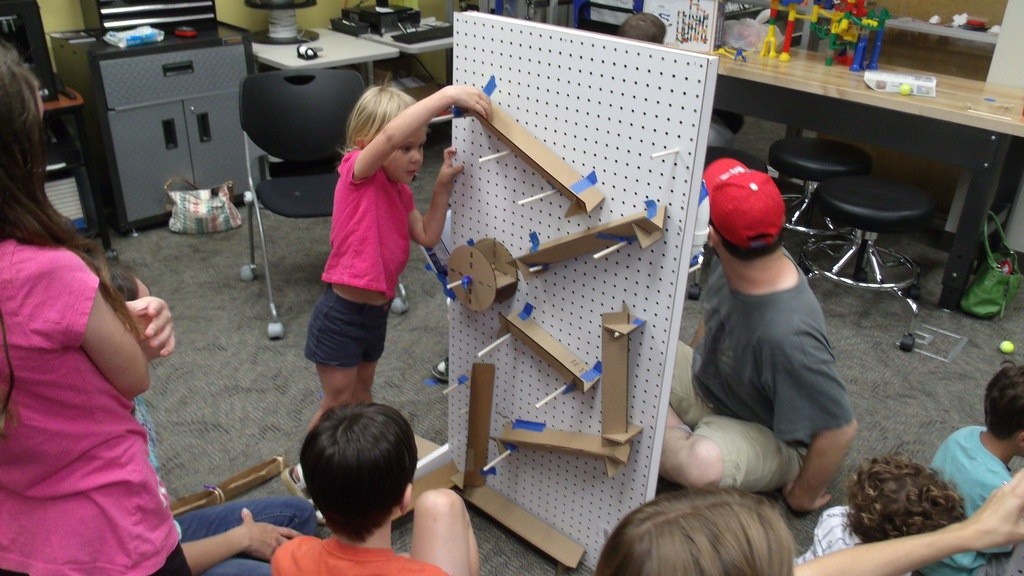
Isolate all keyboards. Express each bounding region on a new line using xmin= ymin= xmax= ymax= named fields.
xmin=391 ymin=24 xmax=453 ymax=45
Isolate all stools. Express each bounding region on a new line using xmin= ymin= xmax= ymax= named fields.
xmin=765 ymin=138 xmax=870 ymax=234
xmin=799 ymin=175 xmax=931 ymax=352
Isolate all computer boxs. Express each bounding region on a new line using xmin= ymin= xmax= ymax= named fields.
xmin=342 ymin=5 xmax=420 ymax=34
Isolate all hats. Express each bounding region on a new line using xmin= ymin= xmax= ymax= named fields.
xmin=703 ymin=158 xmax=786 ymax=250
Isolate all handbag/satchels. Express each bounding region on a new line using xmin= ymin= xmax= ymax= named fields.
xmin=959 ymin=209 xmax=1022 ymax=319
xmin=163 ymin=177 xmax=242 ymax=234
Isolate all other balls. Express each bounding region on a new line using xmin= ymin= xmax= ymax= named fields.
xmin=900 ymin=84 xmax=910 ymax=95
xmin=1000 ymin=340 xmax=1015 ymax=353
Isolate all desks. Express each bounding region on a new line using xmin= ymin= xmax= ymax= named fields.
xmin=329 ymin=19 xmax=453 ymax=123
xmin=250 ymin=27 xmax=399 ymax=85
xmin=712 ymin=42 xmax=1024 ymax=312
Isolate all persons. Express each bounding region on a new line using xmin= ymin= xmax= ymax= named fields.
xmin=105 ymin=268 xmax=318 ymax=576
xmin=908 ymin=361 xmax=1024 ymax=575
xmin=616 ymin=13 xmax=666 ymax=46
xmin=656 ymin=155 xmax=859 ymax=513
xmin=595 ymin=466 xmax=1023 ymax=575
xmin=0 ymin=43 xmax=198 ymax=576
xmin=281 ymin=81 xmax=492 ymax=525
xmin=795 ymin=453 xmax=967 ymax=576
xmin=269 ymin=401 xmax=481 ymax=576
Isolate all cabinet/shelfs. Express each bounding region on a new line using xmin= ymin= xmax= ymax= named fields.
xmin=50 ymin=21 xmax=267 ymax=237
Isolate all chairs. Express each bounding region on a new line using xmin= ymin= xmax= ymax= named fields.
xmin=238 ymin=67 xmax=408 ymax=338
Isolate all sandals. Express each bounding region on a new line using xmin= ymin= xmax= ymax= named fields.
xmin=279 ymin=462 xmax=328 ymax=525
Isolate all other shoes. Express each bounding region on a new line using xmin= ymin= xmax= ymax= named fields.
xmin=429 ymin=355 xmax=449 ymax=382
